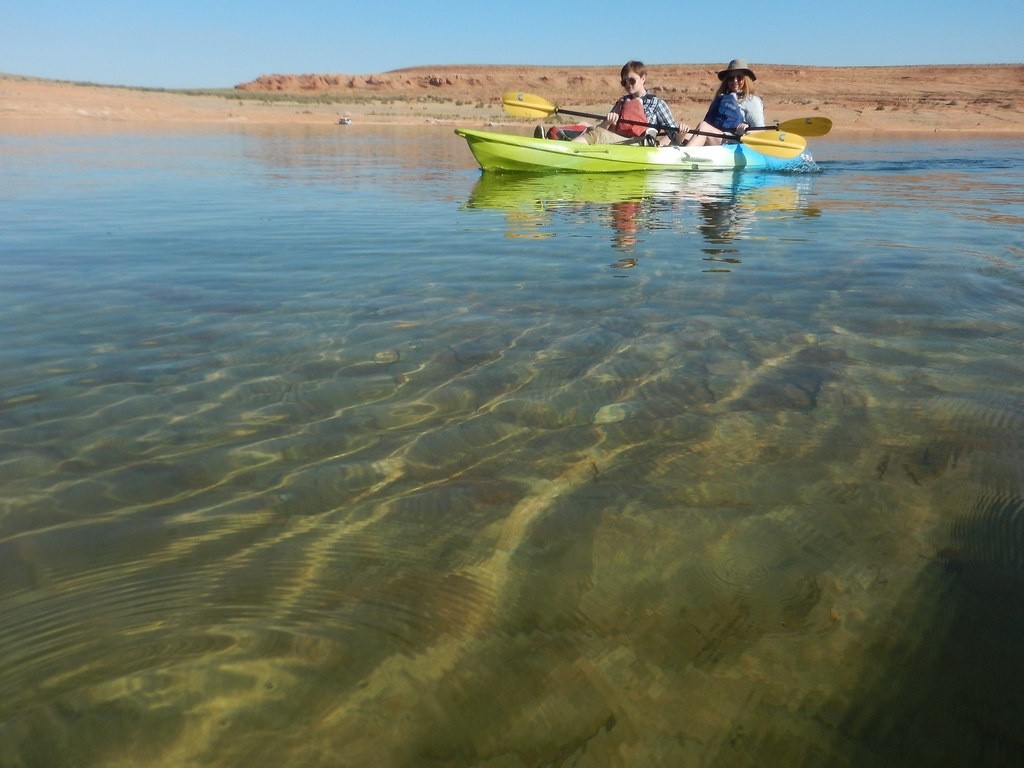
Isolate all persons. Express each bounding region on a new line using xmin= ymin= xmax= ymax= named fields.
xmin=534 ymin=61 xmax=689 ymax=147
xmin=660 ymin=60 xmax=765 ymax=147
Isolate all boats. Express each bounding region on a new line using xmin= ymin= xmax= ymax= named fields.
xmin=463 ymin=172 xmax=818 ymax=210
xmin=453 ymin=125 xmax=824 ymax=174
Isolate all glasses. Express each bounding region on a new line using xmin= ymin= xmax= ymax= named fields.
xmin=727 ymin=75 xmax=744 ymax=82
xmin=621 ymin=77 xmax=640 ymax=87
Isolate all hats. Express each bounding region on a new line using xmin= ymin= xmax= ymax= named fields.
xmin=718 ymin=60 xmax=756 ymax=81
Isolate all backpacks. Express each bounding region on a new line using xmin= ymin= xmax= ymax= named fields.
xmin=609 ymin=94 xmax=656 ymax=136
xmin=702 ymin=91 xmax=744 ymax=133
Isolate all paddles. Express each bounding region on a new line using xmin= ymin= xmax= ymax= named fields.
xmin=502 ymin=91 xmax=807 ymax=160
xmin=719 ymin=117 xmax=833 ymax=138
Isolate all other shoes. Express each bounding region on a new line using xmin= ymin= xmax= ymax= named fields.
xmin=534 ymin=125 xmax=545 ymax=139
